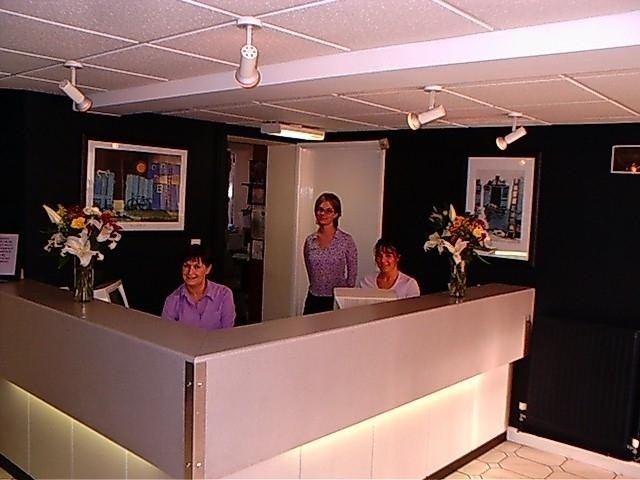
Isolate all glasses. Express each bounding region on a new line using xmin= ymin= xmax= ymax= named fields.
xmin=317 ymin=206 xmax=335 ymax=216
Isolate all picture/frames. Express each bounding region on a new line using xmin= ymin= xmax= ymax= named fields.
xmin=0 ymin=226 xmax=24 ymax=281
xmin=82 ymin=138 xmax=189 ymax=234
xmin=461 ymin=151 xmax=543 ymax=269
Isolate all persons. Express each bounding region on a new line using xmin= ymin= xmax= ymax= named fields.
xmin=357 ymin=238 xmax=421 ymax=305
xmin=160 ymin=244 xmax=238 ymax=332
xmin=303 ymin=192 xmax=359 ymax=316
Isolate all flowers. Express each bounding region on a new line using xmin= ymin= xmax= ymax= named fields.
xmin=41 ymin=203 xmax=122 ymax=299
xmin=422 ymin=203 xmax=497 ymax=294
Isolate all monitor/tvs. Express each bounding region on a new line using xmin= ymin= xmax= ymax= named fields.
xmin=332 ymin=287 xmax=399 ymax=311
xmin=93 ymin=279 xmax=130 ymax=309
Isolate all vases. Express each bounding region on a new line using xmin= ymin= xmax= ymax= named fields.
xmin=73 ymin=257 xmax=95 ymax=302
xmin=447 ymin=253 xmax=468 ymax=298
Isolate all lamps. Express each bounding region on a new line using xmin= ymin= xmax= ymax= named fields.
xmin=406 ymin=85 xmax=446 ymax=132
xmin=496 ymin=112 xmax=528 ymax=152
xmin=58 ymin=61 xmax=93 ymax=113
xmin=260 ymin=121 xmax=325 ymax=142
xmin=232 ymin=17 xmax=264 ymax=89
